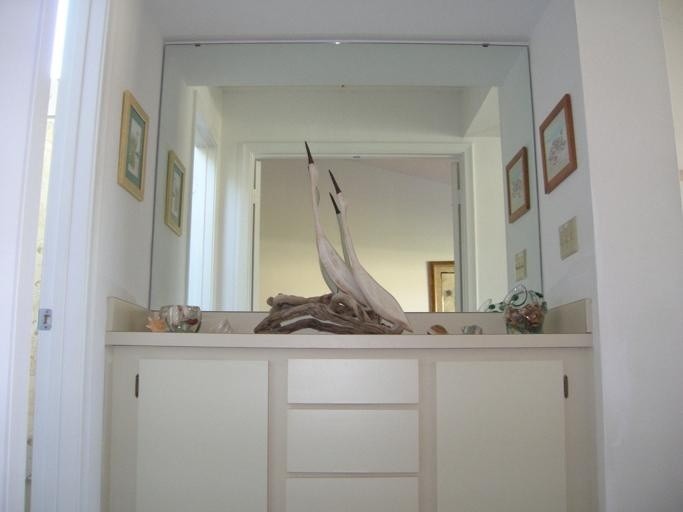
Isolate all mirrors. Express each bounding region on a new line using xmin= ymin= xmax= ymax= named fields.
xmin=150 ymin=42 xmax=542 ymax=313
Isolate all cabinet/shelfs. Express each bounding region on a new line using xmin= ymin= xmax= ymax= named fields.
xmin=136 ymin=359 xmax=268 ymax=512
xmin=286 ymin=359 xmax=419 ymax=512
xmin=435 ymin=360 xmax=568 ymax=512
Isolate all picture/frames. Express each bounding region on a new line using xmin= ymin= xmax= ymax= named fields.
xmin=506 ymin=147 xmax=530 ymax=224
xmin=427 ymin=261 xmax=456 ymax=312
xmin=117 ymin=90 xmax=150 ymax=201
xmin=164 ymin=151 xmax=187 ymax=236
xmin=538 ymin=93 xmax=578 ymax=194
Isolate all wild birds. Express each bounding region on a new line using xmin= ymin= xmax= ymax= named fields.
xmin=303 ymin=140 xmax=414 ymax=333
